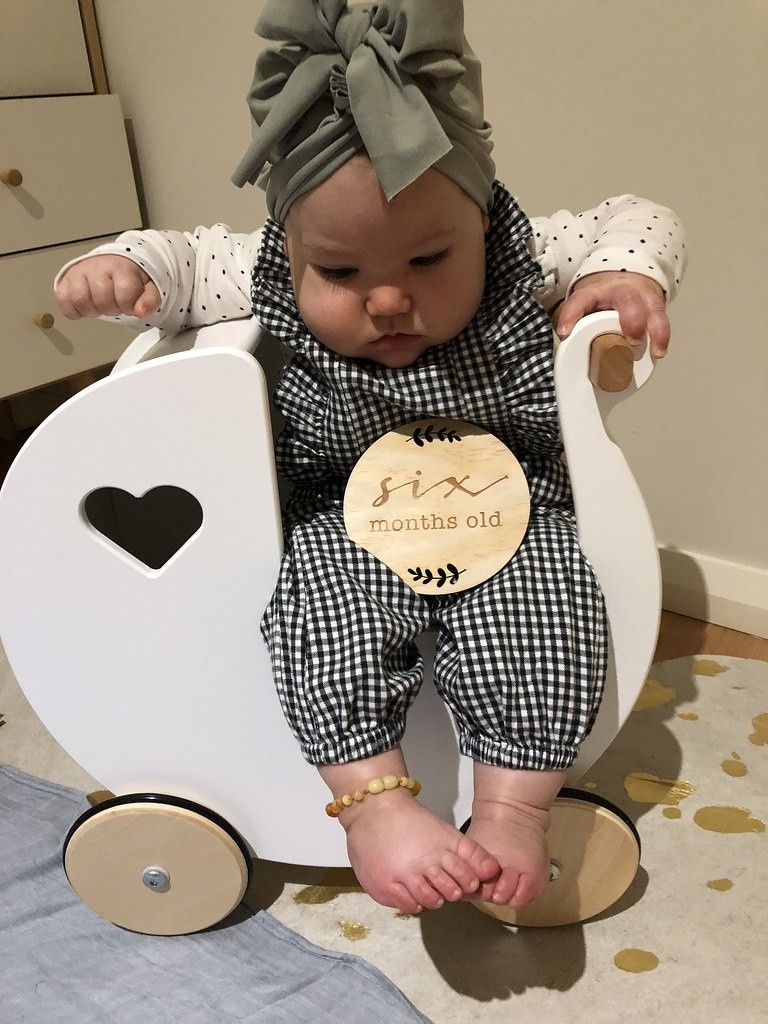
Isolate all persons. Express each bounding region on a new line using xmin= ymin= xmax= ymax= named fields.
xmin=52 ymin=1 xmax=686 ymax=914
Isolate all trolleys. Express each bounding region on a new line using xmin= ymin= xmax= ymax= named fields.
xmin=0 ymin=311 xmax=666 ymax=936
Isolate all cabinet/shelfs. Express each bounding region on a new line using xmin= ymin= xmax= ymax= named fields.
xmin=0 ymin=0 xmax=150 ymax=441
